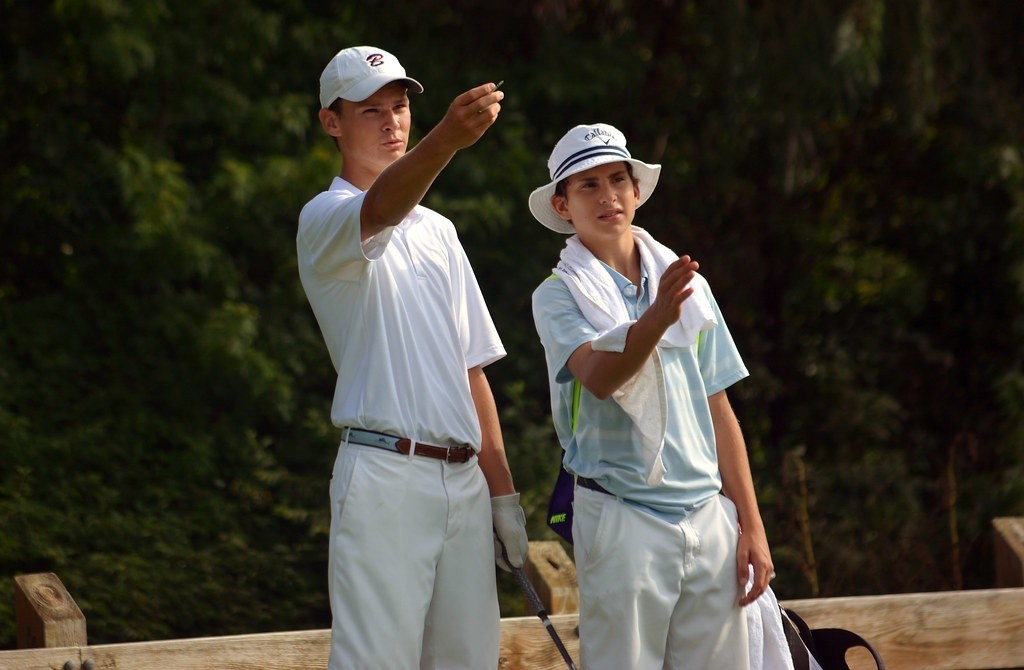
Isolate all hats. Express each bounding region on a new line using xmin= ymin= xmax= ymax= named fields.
xmin=319 ymin=45 xmax=424 ymax=111
xmin=528 ymin=123 xmax=662 ymax=234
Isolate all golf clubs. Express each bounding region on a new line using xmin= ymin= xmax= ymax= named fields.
xmin=498 ymin=531 xmax=576 ymax=670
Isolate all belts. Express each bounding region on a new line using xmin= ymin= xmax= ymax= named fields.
xmin=577 ymin=475 xmax=616 ymax=496
xmin=340 ymin=427 xmax=475 ymax=465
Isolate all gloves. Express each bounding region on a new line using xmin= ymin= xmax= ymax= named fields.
xmin=491 ymin=493 xmax=529 ymax=573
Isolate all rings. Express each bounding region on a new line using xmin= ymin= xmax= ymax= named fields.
xmin=770 ymin=572 xmax=776 ymax=579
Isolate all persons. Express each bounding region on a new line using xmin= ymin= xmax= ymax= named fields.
xmin=294 ymin=45 xmax=529 ymax=670
xmin=529 ymin=124 xmax=775 ymax=670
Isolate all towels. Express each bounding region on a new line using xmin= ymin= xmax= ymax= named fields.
xmin=548 ymin=221 xmax=721 ymax=490
